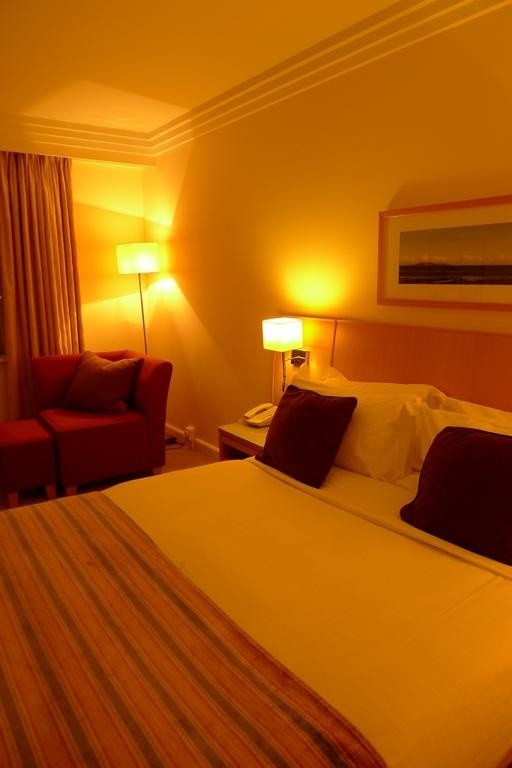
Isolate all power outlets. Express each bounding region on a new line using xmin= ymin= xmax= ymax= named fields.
xmin=184 ymin=426 xmax=196 ymax=440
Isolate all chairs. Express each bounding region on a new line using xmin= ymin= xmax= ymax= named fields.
xmin=22 ymin=351 xmax=173 ymax=497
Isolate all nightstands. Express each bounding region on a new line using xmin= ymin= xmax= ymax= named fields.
xmin=217 ymin=420 xmax=270 ymax=461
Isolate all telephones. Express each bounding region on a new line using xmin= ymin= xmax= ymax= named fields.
xmin=238 ymin=402 xmax=278 ymax=427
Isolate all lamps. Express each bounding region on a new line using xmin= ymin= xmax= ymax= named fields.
xmin=114 ymin=241 xmax=161 ymax=356
xmin=262 ymin=317 xmax=303 ymax=392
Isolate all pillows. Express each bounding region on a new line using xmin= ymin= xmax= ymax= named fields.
xmin=65 ymin=351 xmax=144 ymax=413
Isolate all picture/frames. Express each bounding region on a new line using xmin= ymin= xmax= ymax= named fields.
xmin=376 ymin=195 xmax=512 ymax=312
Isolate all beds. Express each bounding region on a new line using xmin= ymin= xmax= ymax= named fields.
xmin=0 ymin=316 xmax=512 ymax=768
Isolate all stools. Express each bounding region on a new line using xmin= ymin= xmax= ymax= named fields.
xmin=0 ymin=418 xmax=57 ymax=507
